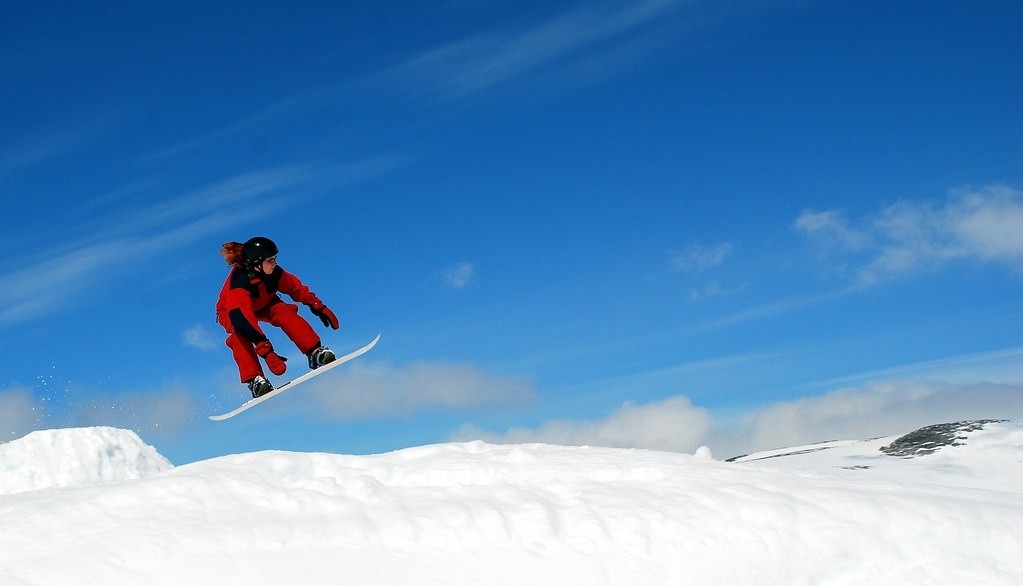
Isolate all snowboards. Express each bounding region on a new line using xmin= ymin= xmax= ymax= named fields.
xmin=208 ymin=333 xmax=382 ymax=421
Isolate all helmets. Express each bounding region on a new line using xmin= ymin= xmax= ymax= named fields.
xmin=242 ymin=237 xmax=279 ymax=262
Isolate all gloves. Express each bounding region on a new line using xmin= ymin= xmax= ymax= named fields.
xmin=253 ymin=339 xmax=288 ymax=376
xmin=310 ymin=300 xmax=339 ymax=330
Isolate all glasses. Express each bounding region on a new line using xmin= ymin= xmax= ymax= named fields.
xmin=264 ymin=256 xmax=277 ymax=263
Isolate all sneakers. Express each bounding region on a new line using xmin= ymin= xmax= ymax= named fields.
xmin=247 ymin=375 xmax=273 ymax=397
xmin=308 ymin=346 xmax=335 ymax=369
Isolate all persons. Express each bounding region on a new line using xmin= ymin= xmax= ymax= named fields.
xmin=216 ymin=237 xmax=339 ymax=398
